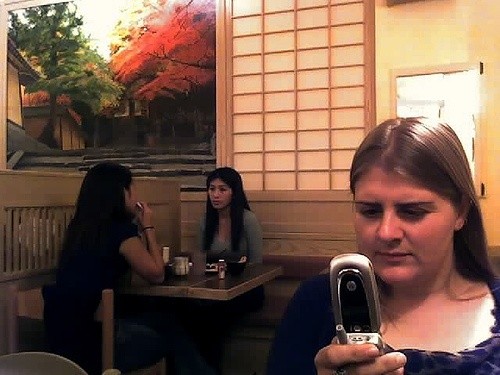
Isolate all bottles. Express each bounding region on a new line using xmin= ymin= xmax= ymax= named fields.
xmin=218 ymin=260 xmax=226 ymax=280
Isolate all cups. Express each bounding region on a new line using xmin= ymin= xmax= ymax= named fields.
xmin=174 ymin=256 xmax=189 ymax=280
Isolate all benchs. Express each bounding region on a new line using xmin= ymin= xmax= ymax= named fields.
xmin=214 ymin=256 xmax=334 ymax=339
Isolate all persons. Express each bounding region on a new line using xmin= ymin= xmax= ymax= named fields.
xmin=267 ymin=116 xmax=500 ymax=375
xmin=40 ymin=161 xmax=216 ymax=375
xmin=170 ymin=168 xmax=263 ymax=375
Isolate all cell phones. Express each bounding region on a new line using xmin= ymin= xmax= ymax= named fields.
xmin=327 ymin=251 xmax=395 ymax=355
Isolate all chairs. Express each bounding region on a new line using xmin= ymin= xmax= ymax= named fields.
xmin=4 ymin=282 xmax=166 ymax=373
